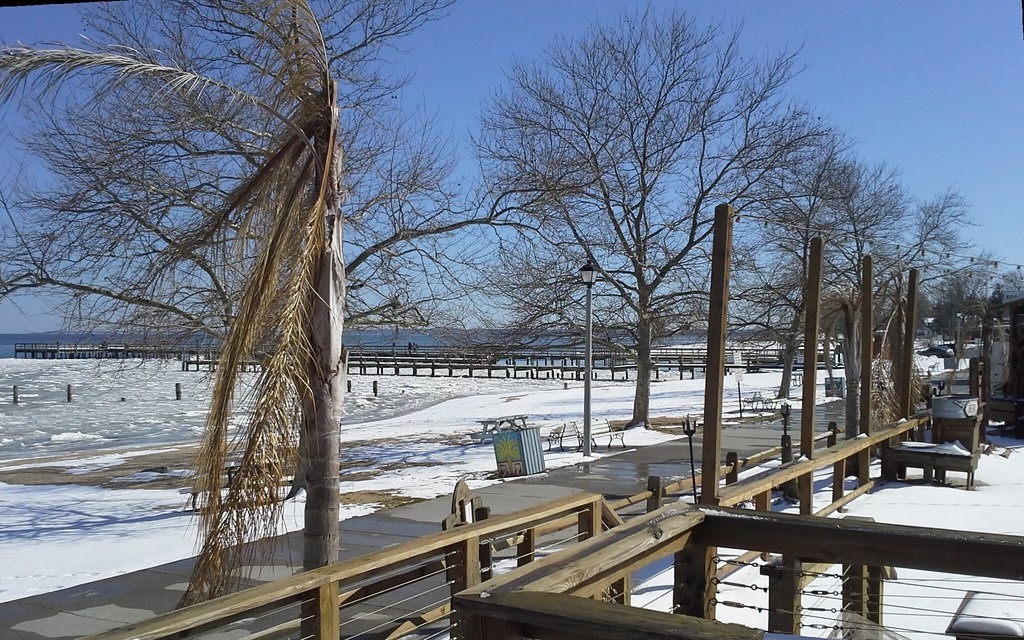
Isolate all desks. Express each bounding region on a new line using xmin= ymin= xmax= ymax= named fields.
xmin=792 ymin=372 xmax=802 ymax=387
xmin=475 ymin=415 xmax=529 ymax=445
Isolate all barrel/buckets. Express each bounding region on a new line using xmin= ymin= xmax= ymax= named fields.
xmin=931 ymin=396 xmax=978 ymax=452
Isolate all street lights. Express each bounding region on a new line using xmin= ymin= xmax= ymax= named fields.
xmin=579 ymin=260 xmax=599 ymax=458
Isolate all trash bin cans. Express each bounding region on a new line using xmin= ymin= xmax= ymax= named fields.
xmin=491 ymin=427 xmax=546 ymax=477
xmin=825 ymin=377 xmax=846 ymax=398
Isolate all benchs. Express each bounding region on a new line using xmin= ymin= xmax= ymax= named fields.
xmin=464 ymin=428 xmax=501 ymax=436
xmin=759 ymin=390 xmax=788 ymax=412
xmin=506 ymin=423 xmax=543 ymax=437
xmin=547 ymin=417 xmax=596 ymax=451
xmin=574 ymin=419 xmax=626 ymax=451
xmin=742 ymin=389 xmax=770 ymax=411
xmin=178 ymin=460 xmax=296 ymax=514
